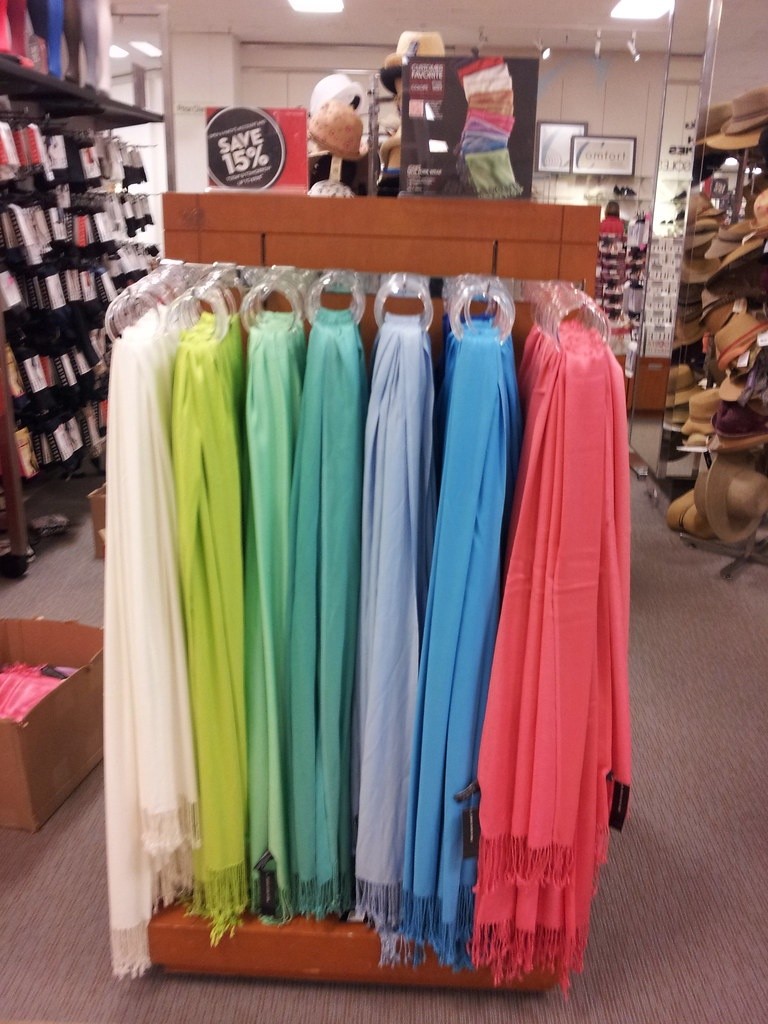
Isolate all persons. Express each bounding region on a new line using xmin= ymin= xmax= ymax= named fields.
xmin=600 ymin=202 xmax=624 ymax=233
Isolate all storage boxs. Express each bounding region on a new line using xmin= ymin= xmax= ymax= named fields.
xmin=0 ymin=618 xmax=103 ymax=833
xmin=86 ymin=482 xmax=106 ymax=557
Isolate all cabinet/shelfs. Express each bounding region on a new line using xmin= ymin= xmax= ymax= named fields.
xmin=671 ymin=149 xmax=768 ymax=576
xmin=1 ymin=57 xmax=166 ymax=570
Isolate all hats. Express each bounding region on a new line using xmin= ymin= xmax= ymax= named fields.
xmin=308 ymin=30 xmax=447 ymax=199
xmin=664 ymin=82 xmax=768 ymax=547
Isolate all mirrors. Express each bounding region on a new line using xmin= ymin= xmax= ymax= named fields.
xmin=629 ymin=0 xmax=717 ymax=478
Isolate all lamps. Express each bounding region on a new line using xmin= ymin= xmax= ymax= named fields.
xmin=473 ymin=28 xmax=488 ymax=53
xmin=531 ymin=28 xmax=551 ymax=60
xmin=627 ymin=29 xmax=640 ymax=61
xmin=593 ymin=31 xmax=603 ymax=61
